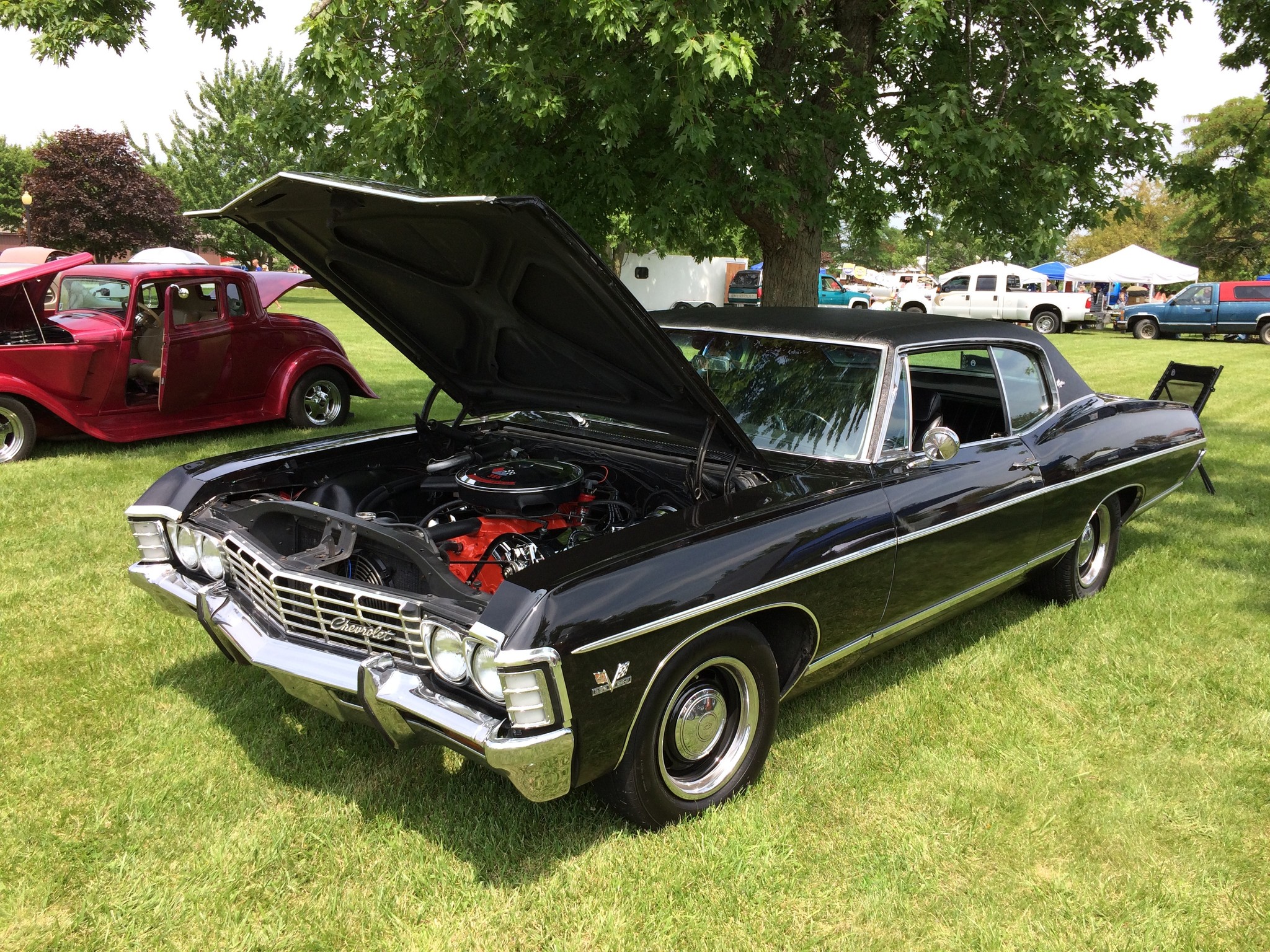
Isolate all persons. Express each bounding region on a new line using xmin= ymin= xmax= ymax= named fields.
xmin=1046 ymin=277 xmax=1058 ymax=293
xmin=1074 ymin=285 xmax=1086 ymax=330
xmin=1167 ymin=290 xmax=1178 ymax=304
xmin=1154 ymin=288 xmax=1166 ymax=302
xmin=1192 ymin=289 xmax=1211 ymax=341
xmin=1118 ymin=286 xmax=1130 ymax=305
xmin=251 ymin=259 xmax=282 ymax=310
xmin=1090 ymin=285 xmax=1106 ymax=310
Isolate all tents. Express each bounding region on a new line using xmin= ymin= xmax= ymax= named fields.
xmin=1025 ymin=261 xmax=1074 ymax=283
xmin=1062 ymin=244 xmax=1198 ymax=305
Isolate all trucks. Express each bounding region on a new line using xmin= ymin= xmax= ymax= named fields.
xmin=842 ymin=273 xmax=941 ymax=300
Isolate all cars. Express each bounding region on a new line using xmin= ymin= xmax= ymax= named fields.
xmin=0 ymin=244 xmax=81 ymax=311
xmin=123 ymin=170 xmax=1212 ymax=832
xmin=1 ymin=252 xmax=381 ymax=465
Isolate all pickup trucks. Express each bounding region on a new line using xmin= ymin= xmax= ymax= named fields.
xmin=1113 ymin=280 xmax=1270 ymax=344
xmin=895 ymin=260 xmax=1107 ymax=336
xmin=728 ymin=267 xmax=871 ymax=311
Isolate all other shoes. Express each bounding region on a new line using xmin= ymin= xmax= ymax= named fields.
xmin=1203 ymin=337 xmax=1210 ymax=340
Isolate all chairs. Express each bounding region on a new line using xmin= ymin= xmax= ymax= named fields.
xmin=892 ymin=389 xmax=944 ymax=452
xmin=139 ymin=312 xmax=218 ymax=383
xmin=128 ymin=308 xmax=199 ymax=378
xmin=1149 ymin=360 xmax=1224 ymax=495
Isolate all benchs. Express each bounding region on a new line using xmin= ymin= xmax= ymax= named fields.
xmin=942 ymin=396 xmax=1005 ymax=444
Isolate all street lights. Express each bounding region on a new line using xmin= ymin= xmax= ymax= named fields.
xmin=921 ymin=229 xmax=934 ymax=280
xmin=21 ymin=191 xmax=33 ymax=248
